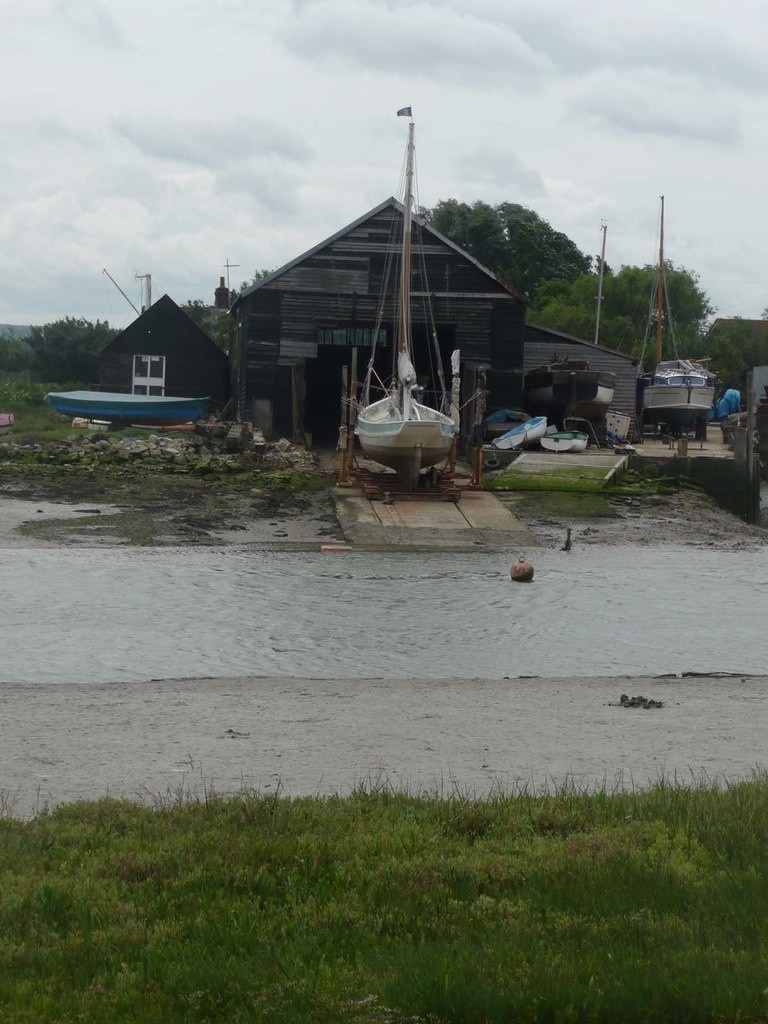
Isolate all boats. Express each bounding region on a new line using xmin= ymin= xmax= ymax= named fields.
xmin=41 ymin=388 xmax=215 ymax=428
xmin=493 ymin=415 xmax=548 ymax=450
xmin=539 ymin=429 xmax=590 ymax=453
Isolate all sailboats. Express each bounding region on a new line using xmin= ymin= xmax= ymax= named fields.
xmin=356 ymin=122 xmax=454 ymax=475
xmin=636 ymin=195 xmax=716 ymax=423
